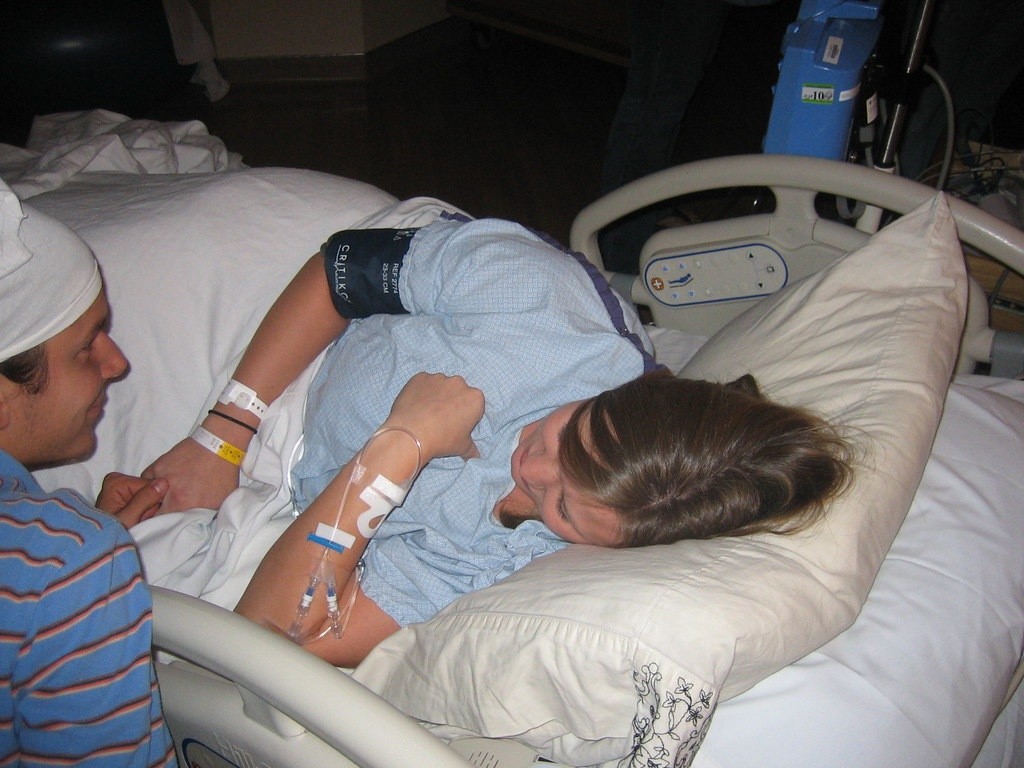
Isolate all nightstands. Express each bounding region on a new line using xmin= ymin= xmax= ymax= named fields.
xmin=902 ymin=129 xmax=1023 ymax=375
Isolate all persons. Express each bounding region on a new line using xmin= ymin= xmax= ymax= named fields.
xmin=139 ymin=219 xmax=855 ymax=669
xmin=0 ymin=177 xmax=177 ymax=768
xmin=595 ymin=0 xmax=773 ymax=324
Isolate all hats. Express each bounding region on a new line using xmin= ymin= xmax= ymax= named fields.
xmin=0 ymin=178 xmax=102 ymax=363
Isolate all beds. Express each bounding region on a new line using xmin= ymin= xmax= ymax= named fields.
xmin=0 ymin=108 xmax=1024 ymax=768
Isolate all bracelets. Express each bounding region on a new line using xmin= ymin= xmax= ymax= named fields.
xmin=190 ymin=425 xmax=246 ymax=466
xmin=217 ymin=378 xmax=269 ymax=420
xmin=208 ymin=410 xmax=258 ymax=434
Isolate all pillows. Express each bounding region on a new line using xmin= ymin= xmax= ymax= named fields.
xmin=349 ymin=191 xmax=969 ymax=768
